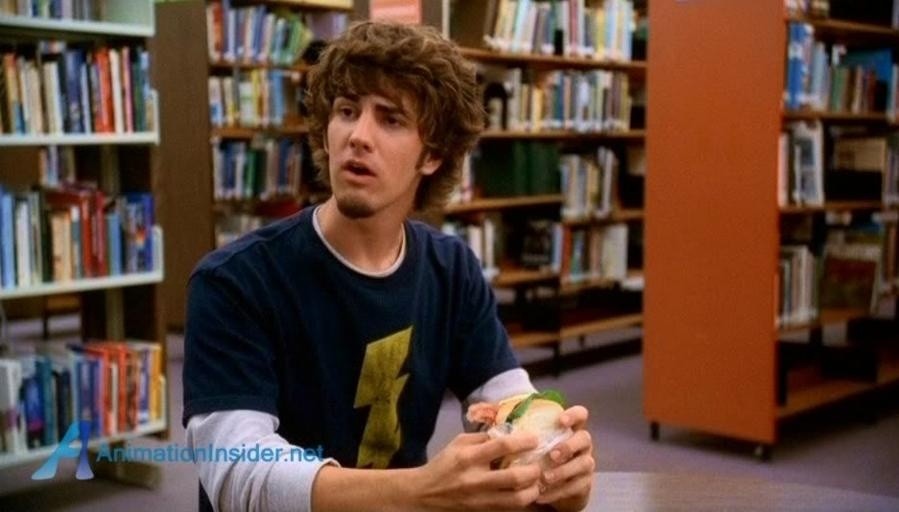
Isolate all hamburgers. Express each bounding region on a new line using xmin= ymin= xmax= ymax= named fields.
xmin=465 ymin=390 xmax=573 ymax=504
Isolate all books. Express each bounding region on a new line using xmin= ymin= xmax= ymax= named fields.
xmin=1 ymin=1 xmax=161 ymax=457
xmin=206 ymin=0 xmax=316 ymax=248
xmin=441 ymin=0 xmax=635 ymax=305
xmin=778 ymin=1 xmax=899 ymax=330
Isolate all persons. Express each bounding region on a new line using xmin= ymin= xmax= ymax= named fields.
xmin=183 ymin=20 xmax=595 ymax=511
